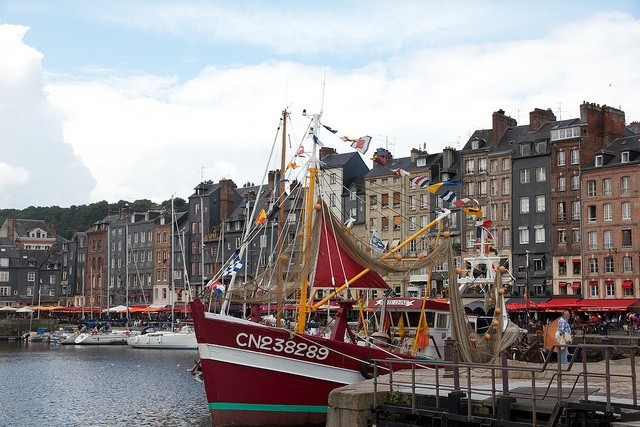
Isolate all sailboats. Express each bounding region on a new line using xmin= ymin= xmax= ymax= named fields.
xmin=190 ymin=111 xmax=527 ymax=425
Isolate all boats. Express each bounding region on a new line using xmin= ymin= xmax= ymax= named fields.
xmin=74 ymin=332 xmax=127 ymax=343
xmin=60 ymin=333 xmax=77 ymax=343
xmin=127 ymin=331 xmax=198 ymax=348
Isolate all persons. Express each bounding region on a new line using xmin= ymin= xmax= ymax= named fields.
xmin=583 ymin=312 xmax=639 ymax=334
xmin=554 ymin=308 xmax=571 ymax=368
